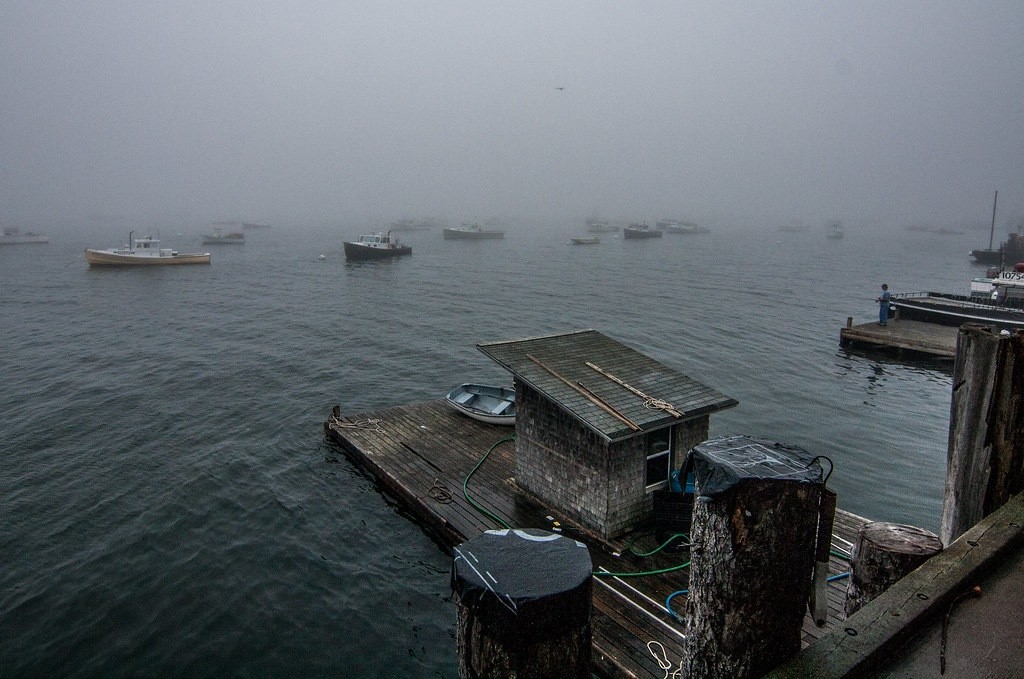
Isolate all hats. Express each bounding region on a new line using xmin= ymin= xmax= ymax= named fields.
xmin=880 ymin=284 xmax=888 ymax=288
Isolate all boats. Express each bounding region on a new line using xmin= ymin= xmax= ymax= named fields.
xmin=827 ymin=221 xmax=845 ymax=239
xmin=85 ymin=231 xmax=211 ymax=265
xmin=343 ymin=230 xmax=412 ymax=259
xmin=200 ymin=232 xmax=244 ymax=243
xmin=588 ymin=220 xmax=621 ymax=231
xmin=443 ymin=223 xmax=504 ymax=240
xmin=670 ymin=221 xmax=709 ymax=232
xmin=777 ymin=221 xmax=813 ymax=231
xmin=0 ymin=226 xmax=48 ymax=243
xmin=571 ymin=237 xmax=600 ymax=244
xmin=624 ymin=223 xmax=663 ymax=238
xmin=445 ymin=383 xmax=516 ymax=425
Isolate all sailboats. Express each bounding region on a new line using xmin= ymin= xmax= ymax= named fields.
xmin=972 ymin=190 xmax=1000 ymax=262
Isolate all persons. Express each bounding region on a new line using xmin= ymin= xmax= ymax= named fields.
xmin=875 ymin=284 xmax=890 ymax=326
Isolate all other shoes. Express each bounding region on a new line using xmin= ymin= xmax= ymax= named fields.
xmin=880 ymin=323 xmax=887 ymax=326
xmin=876 ymin=322 xmax=882 ymax=325
